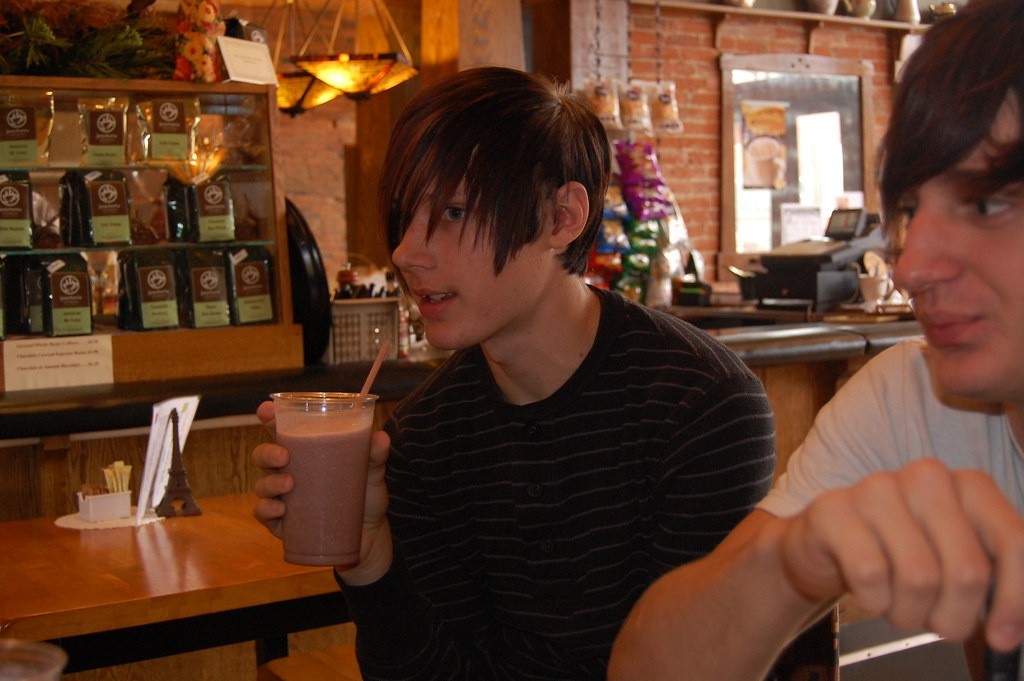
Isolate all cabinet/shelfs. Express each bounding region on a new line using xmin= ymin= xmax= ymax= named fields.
xmin=0 ymin=76 xmax=303 ymax=414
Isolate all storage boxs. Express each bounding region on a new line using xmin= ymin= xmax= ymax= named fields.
xmin=323 ymin=298 xmax=398 ymax=366
xmin=77 ymin=490 xmax=132 ymax=520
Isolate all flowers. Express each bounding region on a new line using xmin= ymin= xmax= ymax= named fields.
xmin=134 ymin=0 xmax=227 ymax=81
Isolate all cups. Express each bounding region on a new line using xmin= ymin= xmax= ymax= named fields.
xmin=271 ymin=391 xmax=378 ymax=567
xmin=0 ymin=639 xmax=68 ymax=681
xmin=859 ymin=273 xmax=892 ymax=302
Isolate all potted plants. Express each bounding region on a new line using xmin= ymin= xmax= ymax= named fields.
xmin=0 ymin=0 xmax=67 ymax=75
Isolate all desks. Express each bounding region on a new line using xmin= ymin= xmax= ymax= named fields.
xmin=0 ymin=491 xmax=349 ymax=666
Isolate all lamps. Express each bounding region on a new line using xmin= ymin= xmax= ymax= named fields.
xmin=293 ymin=2 xmax=415 ymax=100
xmin=255 ymin=1 xmax=343 ymax=117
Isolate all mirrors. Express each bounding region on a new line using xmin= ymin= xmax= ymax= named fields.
xmin=715 ymin=50 xmax=879 ymax=274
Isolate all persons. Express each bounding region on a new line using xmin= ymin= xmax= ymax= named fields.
xmin=252 ymin=67 xmax=775 ymax=681
xmin=599 ymin=0 xmax=1024 ymax=681
